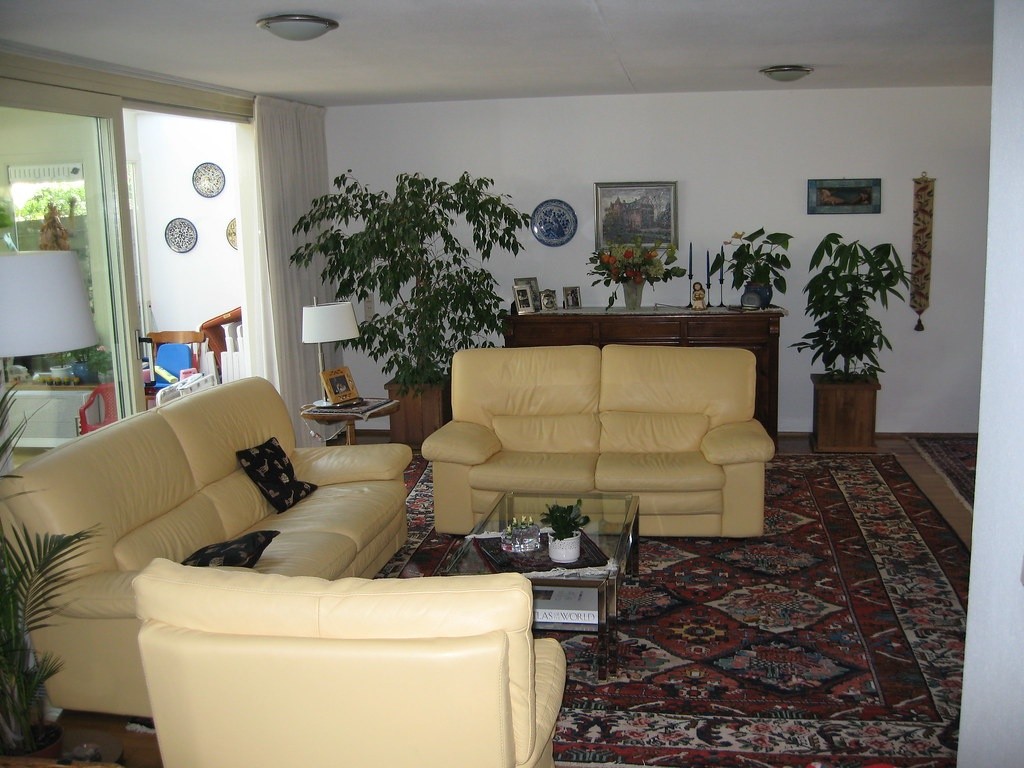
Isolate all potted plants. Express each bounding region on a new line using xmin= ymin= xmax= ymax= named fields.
xmin=255 ymin=15 xmax=337 ymax=41
xmin=541 ymin=499 xmax=590 ymax=561
xmin=0 ymin=368 xmax=105 ymax=759
xmin=783 ymin=233 xmax=911 ymax=452
xmin=284 ymin=165 xmax=532 ymax=447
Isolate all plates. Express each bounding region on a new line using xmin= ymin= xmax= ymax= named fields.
xmin=225 ymin=218 xmax=238 ymax=251
xmin=165 ymin=218 xmax=198 ymax=254
xmin=192 ymin=162 xmax=226 ymax=198
xmin=531 ymin=199 xmax=578 ymax=247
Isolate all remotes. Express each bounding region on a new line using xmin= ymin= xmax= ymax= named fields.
xmin=482 ymin=545 xmax=512 ymax=566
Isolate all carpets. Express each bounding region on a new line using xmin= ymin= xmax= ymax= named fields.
xmin=908 ymin=435 xmax=977 ymax=516
xmin=375 ymin=446 xmax=971 ymax=768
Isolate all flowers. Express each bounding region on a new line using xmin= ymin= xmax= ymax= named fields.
xmin=708 ymin=227 xmax=794 ymax=294
xmin=585 ymin=231 xmax=687 ymax=311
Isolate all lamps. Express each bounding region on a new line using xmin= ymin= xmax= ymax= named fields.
xmin=0 ymin=247 xmax=97 ymax=387
xmin=759 ymin=66 xmax=813 ymax=82
xmin=301 ymin=295 xmax=365 ymax=407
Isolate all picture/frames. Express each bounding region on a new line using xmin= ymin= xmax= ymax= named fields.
xmin=513 ymin=277 xmax=541 ymax=309
xmin=320 ymin=366 xmax=359 ymax=404
xmin=234 ymin=438 xmax=317 ymax=513
xmin=564 ymin=286 xmax=582 ymax=310
xmin=512 ymin=284 xmax=535 ymax=315
xmin=592 ymin=180 xmax=680 ymax=250
xmin=808 ymin=179 xmax=882 ymax=215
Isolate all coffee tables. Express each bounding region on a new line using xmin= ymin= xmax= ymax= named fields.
xmin=436 ymin=490 xmax=640 ymax=683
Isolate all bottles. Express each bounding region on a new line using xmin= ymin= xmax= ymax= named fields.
xmin=40 ymin=376 xmax=81 ymax=386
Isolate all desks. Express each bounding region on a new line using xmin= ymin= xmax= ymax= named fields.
xmin=298 ymin=400 xmax=400 ymax=446
xmin=502 ymin=307 xmax=782 ymax=452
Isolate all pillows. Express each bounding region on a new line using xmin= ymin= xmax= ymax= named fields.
xmin=179 ymin=529 xmax=280 ymax=570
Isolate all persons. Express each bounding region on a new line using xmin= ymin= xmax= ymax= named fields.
xmin=568 ymin=292 xmax=577 ymax=306
xmin=519 ymin=292 xmax=528 ymax=308
xmin=692 ymin=282 xmax=706 ymax=309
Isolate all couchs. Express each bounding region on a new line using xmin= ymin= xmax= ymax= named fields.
xmin=0 ymin=375 xmax=413 ymax=723
xmin=130 ymin=555 xmax=568 ymax=768
xmin=423 ymin=345 xmax=776 ymax=538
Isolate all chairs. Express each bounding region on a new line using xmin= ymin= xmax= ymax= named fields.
xmin=80 ymin=331 xmax=217 ymax=435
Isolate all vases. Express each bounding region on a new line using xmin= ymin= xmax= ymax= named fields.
xmin=741 ymin=280 xmax=774 ymax=309
xmin=622 ymin=279 xmax=645 ymax=311
